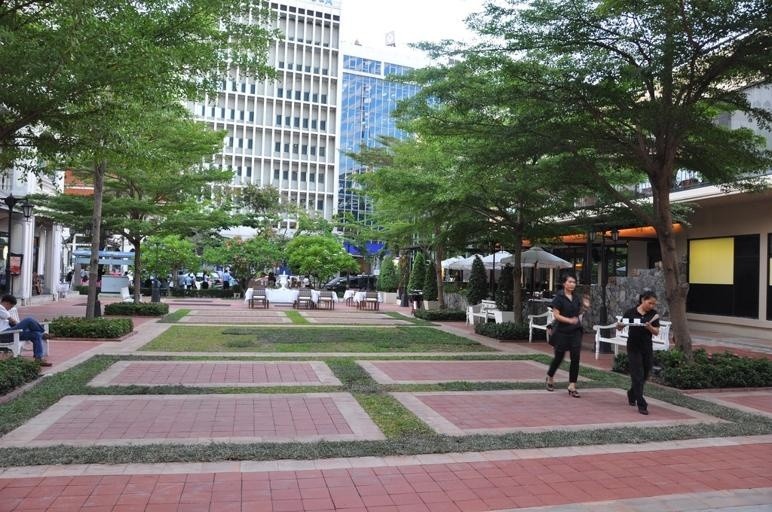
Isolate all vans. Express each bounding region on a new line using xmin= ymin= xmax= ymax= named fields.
xmin=325 ymin=276 xmax=344 ymax=292
xmin=336 ymin=273 xmax=376 ymax=290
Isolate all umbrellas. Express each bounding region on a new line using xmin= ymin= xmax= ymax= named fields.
xmin=440 ymin=244 xmax=573 ymax=298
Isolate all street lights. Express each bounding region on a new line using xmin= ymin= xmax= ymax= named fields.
xmin=488 ymin=240 xmax=501 ymax=301
xmin=0 ymin=192 xmax=35 ymax=295
xmin=149 ymin=240 xmax=166 ymax=302
xmin=85 ymin=222 xmax=114 ymax=316
xmin=587 ymin=225 xmax=620 ymax=353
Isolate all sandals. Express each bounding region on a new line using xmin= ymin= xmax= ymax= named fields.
xmin=545 ymin=377 xmax=554 ymax=391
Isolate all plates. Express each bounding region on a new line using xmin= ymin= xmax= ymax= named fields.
xmin=623 ymin=322 xmax=645 ymax=326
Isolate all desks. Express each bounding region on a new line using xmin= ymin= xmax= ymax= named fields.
xmin=247 ymin=288 xmax=337 ymax=310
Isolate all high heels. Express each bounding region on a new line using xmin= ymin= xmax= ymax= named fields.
xmin=567 ymin=388 xmax=581 ymax=398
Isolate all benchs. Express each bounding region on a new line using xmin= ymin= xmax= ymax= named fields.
xmin=0 ymin=307 xmax=50 ymax=360
xmin=466 ymin=299 xmax=503 ymax=326
xmin=527 ymin=306 xmax=556 ymax=344
xmin=592 ymin=315 xmax=672 ymax=363
xmin=121 ymin=287 xmax=143 ymax=302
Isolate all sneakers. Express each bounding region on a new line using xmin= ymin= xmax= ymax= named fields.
xmin=627 ymin=390 xmax=637 ymax=406
xmin=34 ymin=360 xmax=53 ymax=367
xmin=638 ymin=404 xmax=648 ymax=415
xmin=42 ymin=333 xmax=56 ymax=340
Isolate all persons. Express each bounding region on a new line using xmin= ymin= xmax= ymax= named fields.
xmin=122 ymin=270 xmax=232 ymax=290
xmin=544 ymin=273 xmax=591 ymax=397
xmin=615 ymin=289 xmax=661 ymax=415
xmin=65 ymin=270 xmax=75 ymax=289
xmin=302 ymin=275 xmax=310 ymax=287
xmin=0 ymin=295 xmax=53 ymax=367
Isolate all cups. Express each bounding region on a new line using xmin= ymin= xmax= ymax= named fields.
xmin=622 ymin=318 xmax=629 ymax=323
xmin=633 ymin=318 xmax=640 ymax=323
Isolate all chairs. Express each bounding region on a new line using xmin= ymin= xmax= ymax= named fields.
xmin=251 ymin=285 xmax=269 ymax=309
xmin=296 ymin=286 xmax=335 ymax=310
xmin=346 ymin=287 xmax=380 ymax=311
